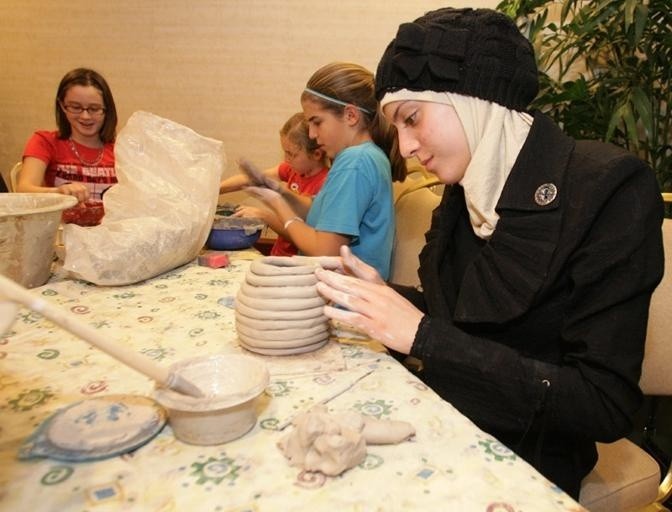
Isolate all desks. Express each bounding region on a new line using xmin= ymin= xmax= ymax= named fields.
xmin=1 ymin=246 xmax=587 ymax=512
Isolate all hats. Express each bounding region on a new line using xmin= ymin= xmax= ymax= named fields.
xmin=376 ymin=8 xmax=538 ymax=112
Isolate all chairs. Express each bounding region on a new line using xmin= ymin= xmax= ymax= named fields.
xmin=580 ymin=192 xmax=672 ymax=511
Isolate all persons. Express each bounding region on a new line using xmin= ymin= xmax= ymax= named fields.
xmin=237 ymin=62 xmax=408 ymax=310
xmin=16 ymin=68 xmax=119 ymax=227
xmin=314 ymin=6 xmax=665 ymax=501
xmin=219 ymin=111 xmax=330 ymax=255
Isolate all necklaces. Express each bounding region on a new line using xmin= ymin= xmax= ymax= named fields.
xmin=72 ymin=136 xmax=104 ymax=167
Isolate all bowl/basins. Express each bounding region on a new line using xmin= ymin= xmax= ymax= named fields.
xmin=62 ymin=200 xmax=105 ymax=226
xmin=0 ymin=192 xmax=79 ymax=291
xmin=205 ymin=217 xmax=262 ymax=250
xmin=152 ymin=350 xmax=272 ymax=446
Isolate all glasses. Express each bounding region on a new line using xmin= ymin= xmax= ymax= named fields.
xmin=62 ymin=102 xmax=106 ymax=115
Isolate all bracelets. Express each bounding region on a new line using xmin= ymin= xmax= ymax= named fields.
xmin=284 ymin=216 xmax=304 ymax=229
